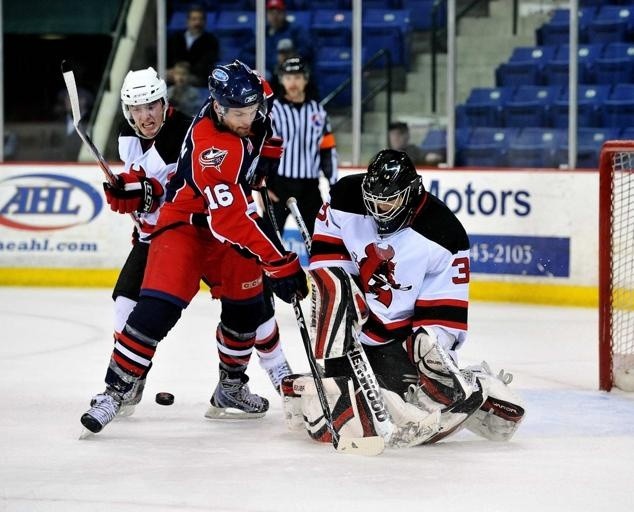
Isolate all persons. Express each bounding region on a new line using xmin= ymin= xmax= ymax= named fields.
xmin=423 ymin=116 xmax=467 ymax=168
xmin=80 ymin=56 xmax=308 ymax=433
xmin=282 ymin=148 xmax=527 ymax=449
xmin=387 ymin=120 xmax=424 ymax=169
xmin=241 ymin=0 xmax=312 ymax=78
xmin=166 ymin=8 xmax=219 ymax=89
xmin=50 ymin=87 xmax=93 ymax=162
xmin=265 ymin=38 xmax=317 ymax=115
xmin=163 ymin=61 xmax=201 ymax=118
xmin=90 ymin=67 xmax=293 ymax=406
xmin=265 ymin=56 xmax=337 ymax=255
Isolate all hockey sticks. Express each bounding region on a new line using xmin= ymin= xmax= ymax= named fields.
xmin=61 ymin=60 xmax=141 ymax=236
xmin=284 ymin=193 xmax=447 ymax=456
xmin=258 ymin=178 xmax=488 ymax=454
xmin=259 ymin=178 xmax=386 ymax=456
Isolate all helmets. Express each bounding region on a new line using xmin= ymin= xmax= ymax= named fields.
xmin=277 ymin=56 xmax=311 ymax=82
xmin=207 ymin=58 xmax=268 ymax=123
xmin=120 ymin=66 xmax=169 ymax=140
xmin=361 ymin=148 xmax=424 ymax=237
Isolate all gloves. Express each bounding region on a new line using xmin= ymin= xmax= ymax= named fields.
xmin=262 ymin=250 xmax=309 ymax=304
xmin=103 ymin=171 xmax=165 ymax=214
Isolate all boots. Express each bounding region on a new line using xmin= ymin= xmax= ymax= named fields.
xmin=88 ymin=377 xmax=146 ymax=409
xmin=256 ymin=351 xmax=294 ymax=397
xmin=204 ymin=363 xmax=271 ymax=415
xmin=78 ymin=385 xmax=124 ymax=433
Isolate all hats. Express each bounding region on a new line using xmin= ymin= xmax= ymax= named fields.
xmin=266 ymin=0 xmax=286 ymax=10
xmin=388 ymin=122 xmax=408 ymax=131
